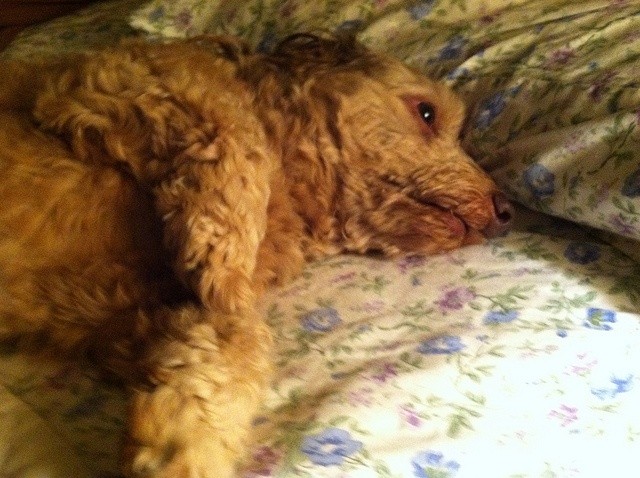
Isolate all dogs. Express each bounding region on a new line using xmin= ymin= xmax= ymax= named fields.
xmin=0 ymin=17 xmax=520 ymax=478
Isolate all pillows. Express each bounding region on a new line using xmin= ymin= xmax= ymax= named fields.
xmin=122 ymin=1 xmax=640 ymax=252
